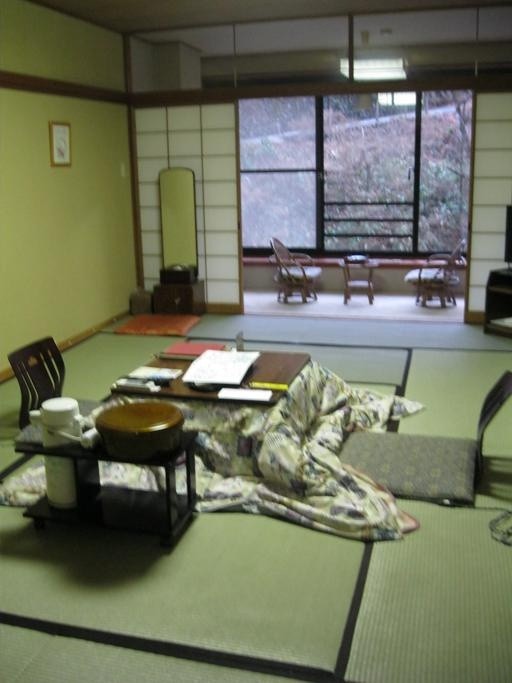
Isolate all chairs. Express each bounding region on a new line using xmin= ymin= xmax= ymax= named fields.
xmin=405 ymin=237 xmax=468 ymax=308
xmin=335 ymin=369 xmax=512 ymax=505
xmin=266 ymin=237 xmax=321 ymax=302
xmin=7 ymin=336 xmax=103 ymax=427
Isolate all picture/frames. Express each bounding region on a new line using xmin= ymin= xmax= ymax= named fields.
xmin=49 ymin=119 xmax=72 ymax=166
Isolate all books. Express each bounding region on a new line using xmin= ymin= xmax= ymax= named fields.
xmin=159 ymin=339 xmax=226 ymax=362
xmin=130 ymin=364 xmax=184 ymax=381
xmin=182 ymin=348 xmax=263 ymax=386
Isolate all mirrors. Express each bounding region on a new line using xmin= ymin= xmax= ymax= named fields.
xmin=157 ymin=165 xmax=199 ymax=284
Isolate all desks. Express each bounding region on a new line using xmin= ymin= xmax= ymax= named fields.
xmin=113 ymin=345 xmax=312 ymax=406
xmin=15 ymin=417 xmax=198 ymax=557
xmin=338 ymin=257 xmax=378 ymax=303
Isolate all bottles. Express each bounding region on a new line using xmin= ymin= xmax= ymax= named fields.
xmin=29 ymin=396 xmax=81 ymax=509
